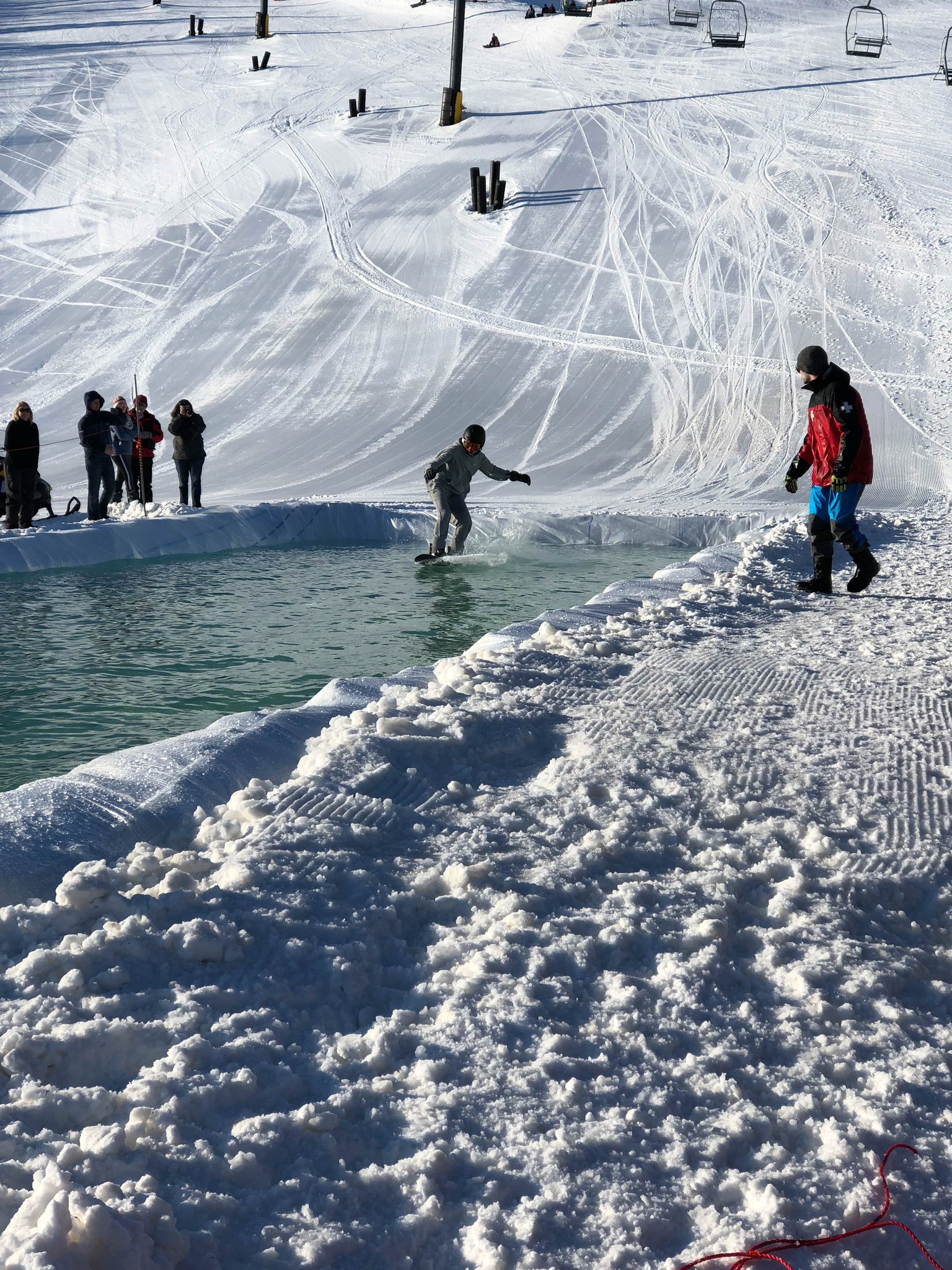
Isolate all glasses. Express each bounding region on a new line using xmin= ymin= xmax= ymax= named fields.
xmin=463 ymin=439 xmax=481 ymax=451
xmin=118 ymin=404 xmax=126 ymax=408
xmin=19 ymin=408 xmax=31 ymax=412
xmin=138 ymin=403 xmax=147 ymax=407
xmin=796 ymin=367 xmax=800 ymax=373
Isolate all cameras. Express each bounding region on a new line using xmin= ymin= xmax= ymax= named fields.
xmin=184 ymin=407 xmax=193 ymax=415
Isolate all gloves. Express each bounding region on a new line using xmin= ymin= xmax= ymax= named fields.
xmin=424 ymin=468 xmax=436 ymax=480
xmin=124 ymin=414 xmax=133 ymax=430
xmin=831 ymin=474 xmax=849 ymax=493
xmin=785 ymin=475 xmax=798 ymax=493
xmin=510 ymin=471 xmax=531 ymax=486
xmin=140 ymin=431 xmax=152 ymax=439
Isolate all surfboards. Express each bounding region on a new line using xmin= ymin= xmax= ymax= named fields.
xmin=415 ymin=554 xmax=457 ymax=566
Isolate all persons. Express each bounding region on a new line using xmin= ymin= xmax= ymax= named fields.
xmin=4 ymin=401 xmax=41 ymax=529
xmin=783 ymin=346 xmax=882 ymax=593
xmin=569 ymin=1 xmax=576 ymax=10
xmin=168 ymin=399 xmax=207 ymax=509
xmin=548 ymin=3 xmax=555 ymax=13
xmin=542 ymin=4 xmax=548 ymax=13
xmin=489 ymin=33 xmax=500 ymax=46
xmin=526 ymin=5 xmax=535 ymax=16
xmin=128 ymin=394 xmax=164 ymax=502
xmin=110 ymin=396 xmax=140 ymax=503
xmin=78 ymin=390 xmax=127 ymax=521
xmin=423 ymin=425 xmax=531 ymax=557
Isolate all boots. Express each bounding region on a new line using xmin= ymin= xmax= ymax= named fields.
xmin=795 ymin=558 xmax=833 ymax=594
xmin=847 ymin=552 xmax=881 ymax=592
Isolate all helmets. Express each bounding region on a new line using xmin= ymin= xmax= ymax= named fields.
xmin=463 ymin=424 xmax=485 ymax=443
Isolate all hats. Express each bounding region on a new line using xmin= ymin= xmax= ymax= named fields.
xmin=797 ymin=346 xmax=828 ymax=375
xmin=113 ymin=396 xmax=126 ymax=408
xmin=133 ymin=394 xmax=147 ymax=409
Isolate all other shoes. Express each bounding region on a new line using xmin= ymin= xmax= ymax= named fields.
xmin=431 ymin=548 xmax=452 ymax=557
xmin=99 ymin=516 xmax=117 ymax=520
